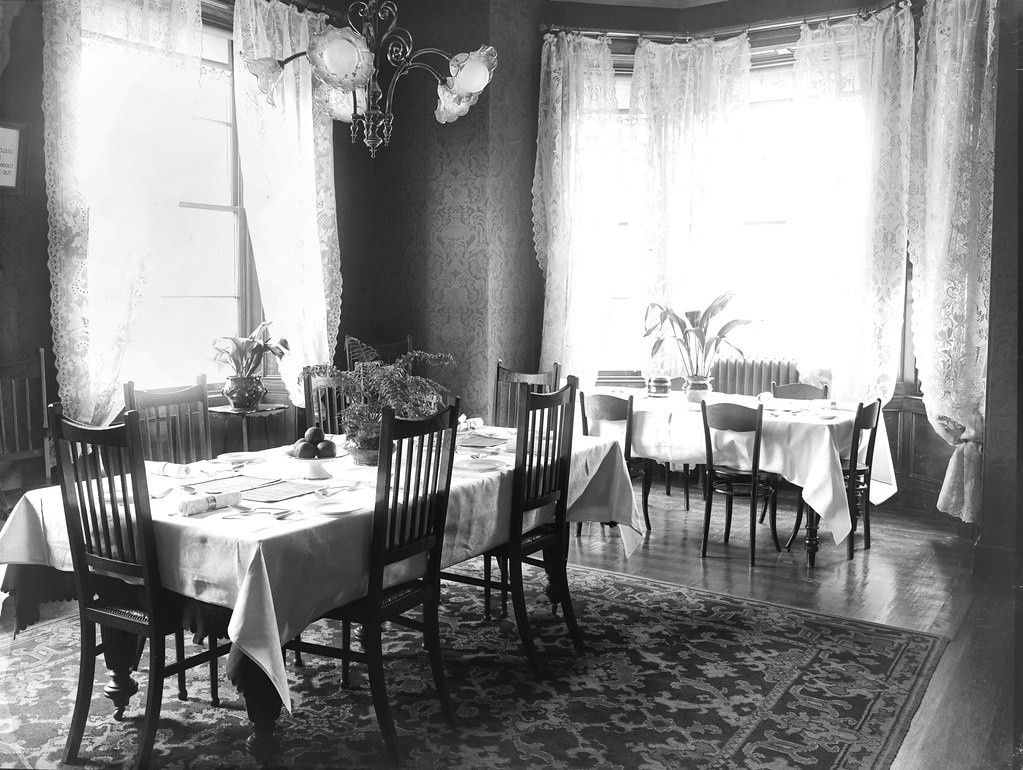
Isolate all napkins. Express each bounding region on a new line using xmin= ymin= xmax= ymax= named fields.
xmin=144 ymin=460 xmax=191 ymax=479
xmin=457 ymin=414 xmax=484 ymax=432
xmin=179 ymin=491 xmax=243 ymax=518
xmin=506 ymin=438 xmax=552 ymax=457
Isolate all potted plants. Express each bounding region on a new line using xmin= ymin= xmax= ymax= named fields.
xmin=643 ymin=288 xmax=753 ymax=404
xmin=210 ymin=319 xmax=291 ymax=412
xmin=296 ymin=333 xmax=458 ymax=467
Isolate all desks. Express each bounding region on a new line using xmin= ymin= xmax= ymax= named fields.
xmin=208 ymin=403 xmax=288 ymax=453
xmin=0 ymin=425 xmax=644 ymax=770
xmin=529 ymin=386 xmax=898 ymax=569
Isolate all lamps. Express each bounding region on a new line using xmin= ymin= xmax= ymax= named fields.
xmin=238 ymin=0 xmax=498 ymax=159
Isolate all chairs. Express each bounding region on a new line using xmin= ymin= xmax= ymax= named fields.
xmin=282 ymin=395 xmax=451 ymax=746
xmin=758 ymin=381 xmax=829 ymax=525
xmin=440 ymin=376 xmax=588 ymax=684
xmin=491 ymin=358 xmax=561 ymax=431
xmin=123 ymin=373 xmax=213 ymax=645
xmin=784 ymin=397 xmax=883 ymax=562
xmin=47 ymin=401 xmax=233 ymax=770
xmin=699 ymin=400 xmax=782 ymax=568
xmin=575 ymin=390 xmax=655 ymax=537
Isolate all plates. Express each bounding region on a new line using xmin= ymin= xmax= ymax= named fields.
xmin=454 ymin=459 xmax=506 ymax=471
xmin=314 ymin=500 xmax=366 ymax=515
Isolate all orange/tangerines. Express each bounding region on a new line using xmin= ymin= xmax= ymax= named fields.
xmin=294 ymin=427 xmax=337 ymax=458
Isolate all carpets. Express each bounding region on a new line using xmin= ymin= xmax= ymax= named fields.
xmin=1 ymin=556 xmax=952 ymax=770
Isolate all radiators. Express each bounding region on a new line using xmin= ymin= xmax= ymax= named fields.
xmin=709 ymin=355 xmax=800 ymax=396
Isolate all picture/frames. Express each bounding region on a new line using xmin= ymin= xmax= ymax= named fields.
xmin=0 ymin=118 xmax=33 ymax=197
